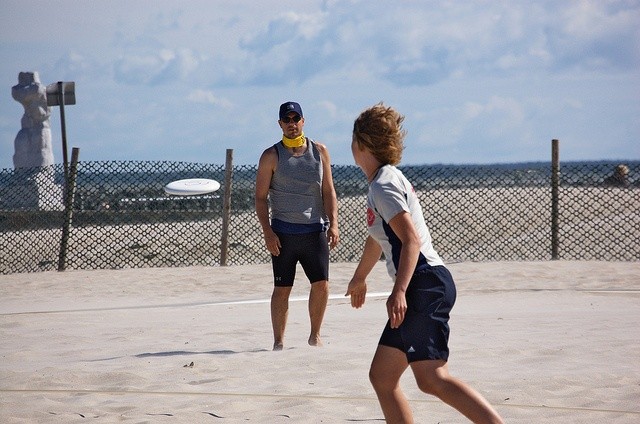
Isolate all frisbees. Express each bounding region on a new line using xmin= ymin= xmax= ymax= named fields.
xmin=165 ymin=178 xmax=221 ymax=195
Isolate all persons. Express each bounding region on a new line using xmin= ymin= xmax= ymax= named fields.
xmin=345 ymin=101 xmax=508 ymax=424
xmin=602 ymin=164 xmax=634 ymax=187
xmin=254 ymin=101 xmax=340 ymax=350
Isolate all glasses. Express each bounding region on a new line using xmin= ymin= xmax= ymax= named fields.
xmin=283 ymin=115 xmax=301 ymax=123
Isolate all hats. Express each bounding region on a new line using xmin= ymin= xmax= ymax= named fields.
xmin=279 ymin=102 xmax=303 ymax=121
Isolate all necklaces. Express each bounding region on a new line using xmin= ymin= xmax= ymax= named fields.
xmin=367 ymin=166 xmax=380 ymax=180
